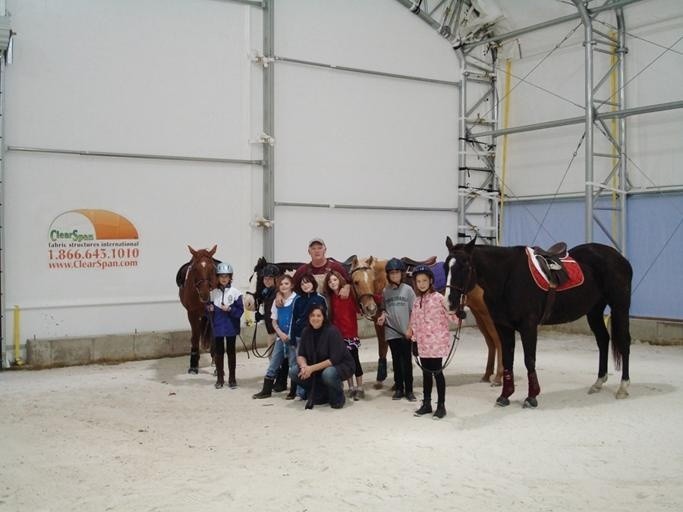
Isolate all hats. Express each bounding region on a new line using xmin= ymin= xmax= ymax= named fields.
xmin=309 ymin=237 xmax=325 ymax=246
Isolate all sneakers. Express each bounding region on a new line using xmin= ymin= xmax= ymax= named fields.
xmin=215 ymin=357 xmax=446 ymax=419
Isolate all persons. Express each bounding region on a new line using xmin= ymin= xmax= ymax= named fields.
xmin=274 ymin=237 xmax=352 ymax=308
xmin=253 ymin=274 xmax=302 ymax=399
xmin=406 ymin=264 xmax=463 ymax=419
xmin=375 ymin=257 xmax=417 ymax=401
xmin=255 ymin=265 xmax=290 ymax=391
xmin=323 ymin=270 xmax=364 ymax=400
xmin=205 ymin=262 xmax=245 ymax=388
xmin=296 ymin=304 xmax=356 ymax=408
xmin=286 ymin=272 xmax=328 ymax=400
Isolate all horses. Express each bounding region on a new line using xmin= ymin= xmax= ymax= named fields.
xmin=347 ymin=256 xmax=504 ymax=391
xmin=243 ymin=257 xmax=307 ymax=311
xmin=179 ymin=244 xmax=225 ymax=376
xmin=444 ymin=234 xmax=633 ymax=408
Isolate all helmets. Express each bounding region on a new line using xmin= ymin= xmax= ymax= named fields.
xmin=385 ymin=258 xmax=405 ymax=271
xmin=217 ymin=262 xmax=233 ymax=274
xmin=412 ymin=264 xmax=432 ymax=274
xmin=262 ymin=264 xmax=280 ymax=277
